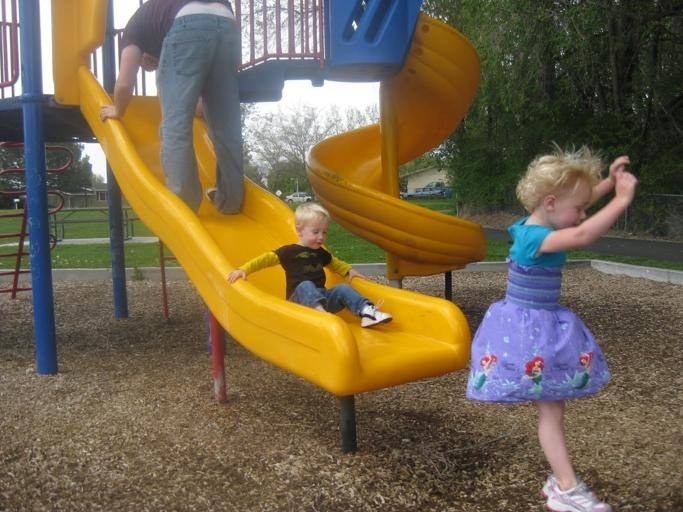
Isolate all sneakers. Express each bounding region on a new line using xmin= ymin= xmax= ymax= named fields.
xmin=361 ymin=305 xmax=392 ymax=328
xmin=540 ymin=474 xmax=612 ymax=512
xmin=206 ymin=187 xmax=218 ymax=202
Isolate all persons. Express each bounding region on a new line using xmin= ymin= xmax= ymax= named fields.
xmin=466 ymin=142 xmax=638 ymax=511
xmin=101 ymin=0 xmax=245 ymax=213
xmin=228 ymin=203 xmax=393 ymax=328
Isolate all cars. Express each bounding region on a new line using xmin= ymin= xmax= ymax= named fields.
xmin=285 ymin=192 xmax=312 ymax=204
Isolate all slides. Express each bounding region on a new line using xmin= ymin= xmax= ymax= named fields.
xmin=80 ymin=69 xmax=471 ymax=395
xmin=303 ymin=12 xmax=487 ymax=281
